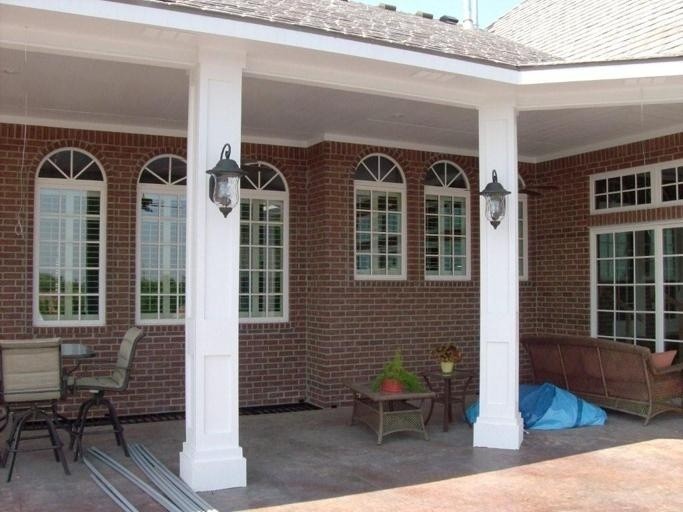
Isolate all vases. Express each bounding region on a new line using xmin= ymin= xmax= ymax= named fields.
xmin=441 ymin=360 xmax=454 ymax=375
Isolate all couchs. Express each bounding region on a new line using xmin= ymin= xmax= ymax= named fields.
xmin=519 ymin=331 xmax=683 ymax=427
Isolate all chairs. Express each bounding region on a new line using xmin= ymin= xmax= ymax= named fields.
xmin=69 ymin=325 xmax=145 ymax=462
xmin=0 ymin=339 xmax=72 ymax=482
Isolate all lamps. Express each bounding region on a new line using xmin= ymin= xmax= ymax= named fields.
xmin=477 ymin=169 xmax=511 ymax=230
xmin=205 ymin=143 xmax=248 ymax=219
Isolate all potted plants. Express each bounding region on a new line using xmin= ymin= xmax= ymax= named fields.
xmin=372 ymin=348 xmax=429 ymax=395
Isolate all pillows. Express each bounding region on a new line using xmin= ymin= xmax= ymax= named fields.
xmin=649 ymin=350 xmax=678 ymax=369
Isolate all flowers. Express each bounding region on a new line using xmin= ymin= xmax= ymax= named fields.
xmin=431 ymin=343 xmax=463 ymax=362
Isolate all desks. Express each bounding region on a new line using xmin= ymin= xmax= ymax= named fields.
xmin=1 ymin=342 xmax=99 ymax=459
